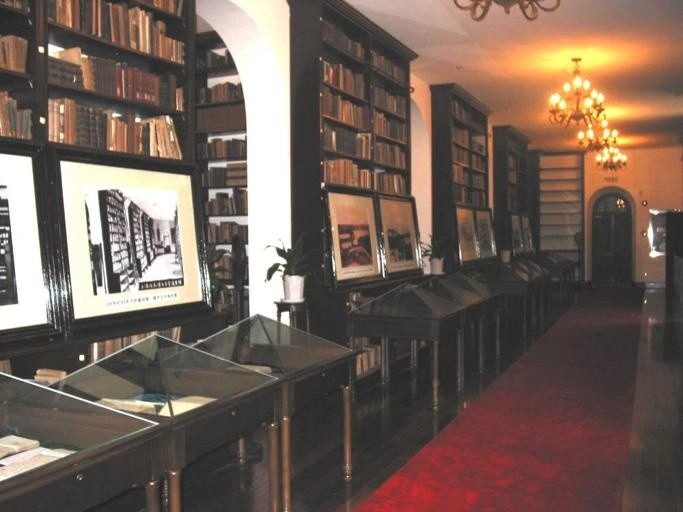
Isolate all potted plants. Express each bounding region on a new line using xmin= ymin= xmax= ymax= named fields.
xmin=260 ymin=231 xmax=323 ymax=301
xmin=422 ymin=233 xmax=453 ymax=274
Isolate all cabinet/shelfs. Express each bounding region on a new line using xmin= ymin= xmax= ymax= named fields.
xmin=428 ymin=81 xmax=493 ymax=274
xmin=494 ymin=124 xmax=532 ymax=257
xmin=285 ymin=0 xmax=415 ymax=385
xmin=0 ymin=2 xmax=45 ymax=142
xmin=535 ymin=148 xmax=584 ymax=284
xmin=44 ymin=0 xmax=195 ymax=162
xmin=196 ymin=32 xmax=247 ymax=321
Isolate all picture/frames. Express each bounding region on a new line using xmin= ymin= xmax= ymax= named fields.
xmin=0 ymin=137 xmax=66 ymax=360
xmin=455 ymin=201 xmax=497 ymax=268
xmin=508 ymin=211 xmax=533 ymax=256
xmin=319 ymin=182 xmax=423 ymax=292
xmin=46 ymin=143 xmax=213 ymax=341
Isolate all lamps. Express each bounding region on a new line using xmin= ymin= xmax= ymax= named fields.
xmin=547 ymin=59 xmax=628 ymax=173
